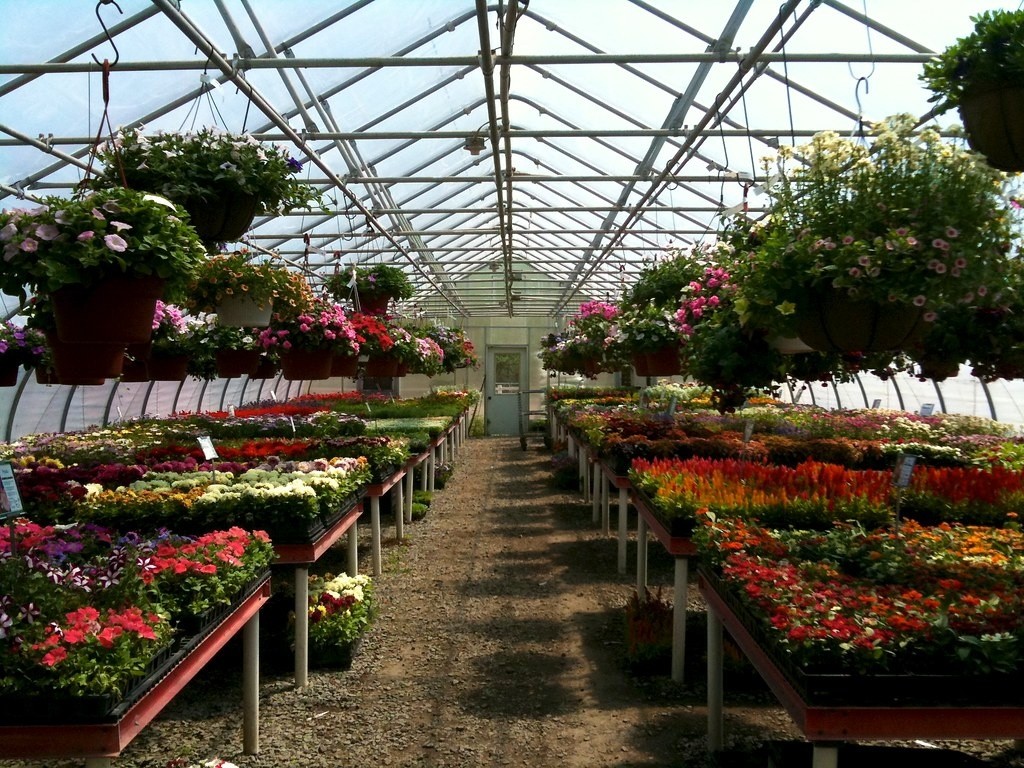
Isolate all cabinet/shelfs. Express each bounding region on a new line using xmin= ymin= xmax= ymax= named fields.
xmin=545 ymin=404 xmax=1024 ymax=768
xmin=1 ymin=402 xmax=469 ymax=748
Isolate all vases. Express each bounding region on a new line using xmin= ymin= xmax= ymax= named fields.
xmin=0 ymin=185 xmax=408 ymax=387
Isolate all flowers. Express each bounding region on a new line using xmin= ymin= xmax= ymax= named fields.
xmin=1 ymin=390 xmax=481 ymax=701
xmin=544 ymin=376 xmax=1024 ymax=674
xmin=535 ymin=9 xmax=1024 ymax=413
xmin=0 ymin=125 xmax=481 ymax=380
